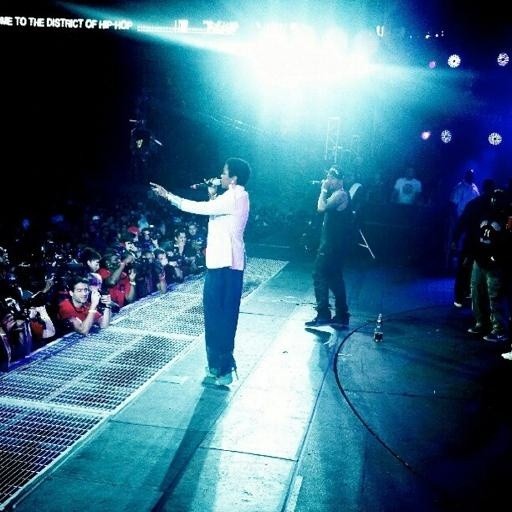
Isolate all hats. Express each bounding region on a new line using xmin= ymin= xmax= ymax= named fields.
xmin=326 ymin=165 xmax=344 ymax=180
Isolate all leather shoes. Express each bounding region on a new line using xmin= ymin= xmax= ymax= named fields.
xmin=305 ymin=315 xmax=332 ymax=326
xmin=330 ymin=316 xmax=349 ymax=327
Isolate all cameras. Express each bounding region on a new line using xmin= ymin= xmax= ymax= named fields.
xmin=0 ymin=231 xmax=207 ymax=323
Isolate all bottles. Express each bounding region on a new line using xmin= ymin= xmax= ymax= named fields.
xmin=374 ymin=313 xmax=384 ymax=343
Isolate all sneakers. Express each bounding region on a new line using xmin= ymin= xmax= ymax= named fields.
xmin=501 ymin=351 xmax=512 ymax=361
xmin=468 ymin=321 xmax=482 ymax=334
xmin=453 ymin=301 xmax=462 ymax=308
xmin=204 ymin=376 xmax=232 ymax=386
xmin=483 ymin=330 xmax=506 ymax=342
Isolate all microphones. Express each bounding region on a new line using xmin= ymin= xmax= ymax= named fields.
xmin=189 ymin=179 xmax=221 ymax=190
xmin=309 ymin=180 xmax=325 ymax=184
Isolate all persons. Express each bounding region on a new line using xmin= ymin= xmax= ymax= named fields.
xmin=451 ymin=169 xmax=480 ymax=209
xmin=149 ymin=157 xmax=250 ymax=386
xmin=389 ymin=165 xmax=423 ymax=207
xmin=0 ymin=201 xmax=207 ymax=366
xmin=451 ymin=179 xmax=512 ymax=361
xmin=304 ymin=164 xmax=351 ymax=330
